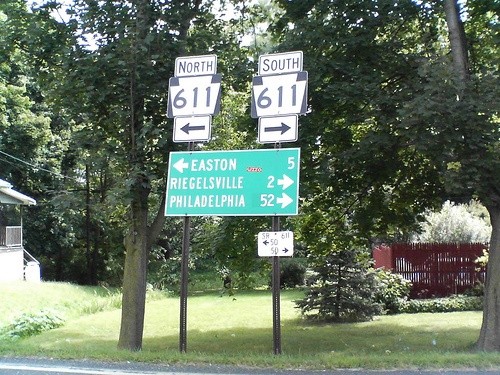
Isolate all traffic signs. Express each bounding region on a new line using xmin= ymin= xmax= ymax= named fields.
xmin=251 ymin=50 xmax=308 ymax=143
xmin=258 ymin=231 xmax=294 ymax=256
xmin=164 ymin=147 xmax=300 ymax=216
xmin=166 ymin=53 xmax=221 ymax=142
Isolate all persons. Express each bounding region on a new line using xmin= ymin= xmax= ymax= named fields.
xmin=219 ymin=272 xmax=233 ymax=298
